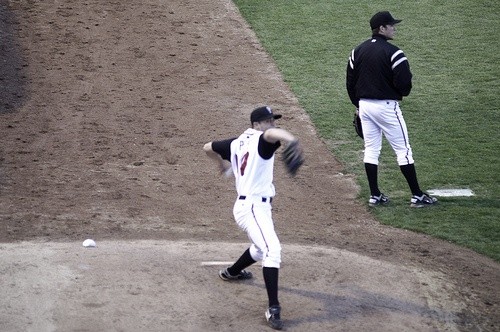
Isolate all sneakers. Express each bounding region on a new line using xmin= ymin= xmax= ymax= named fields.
xmin=218 ymin=266 xmax=252 ymax=281
xmin=265 ymin=305 xmax=282 ymax=330
xmin=411 ymin=193 xmax=438 ymax=207
xmin=369 ymin=193 xmax=390 ymax=207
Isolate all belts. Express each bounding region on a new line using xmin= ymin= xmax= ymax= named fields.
xmin=239 ymin=196 xmax=272 ymax=202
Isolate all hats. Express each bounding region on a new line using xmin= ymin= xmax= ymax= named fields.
xmin=370 ymin=11 xmax=403 ymax=29
xmin=251 ymin=106 xmax=282 ymax=123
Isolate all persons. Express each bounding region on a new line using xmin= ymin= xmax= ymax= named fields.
xmin=346 ymin=9 xmax=438 ymax=208
xmin=203 ymin=106 xmax=306 ymax=330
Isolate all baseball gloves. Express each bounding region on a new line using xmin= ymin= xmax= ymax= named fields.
xmin=353 ymin=112 xmax=364 ymax=138
